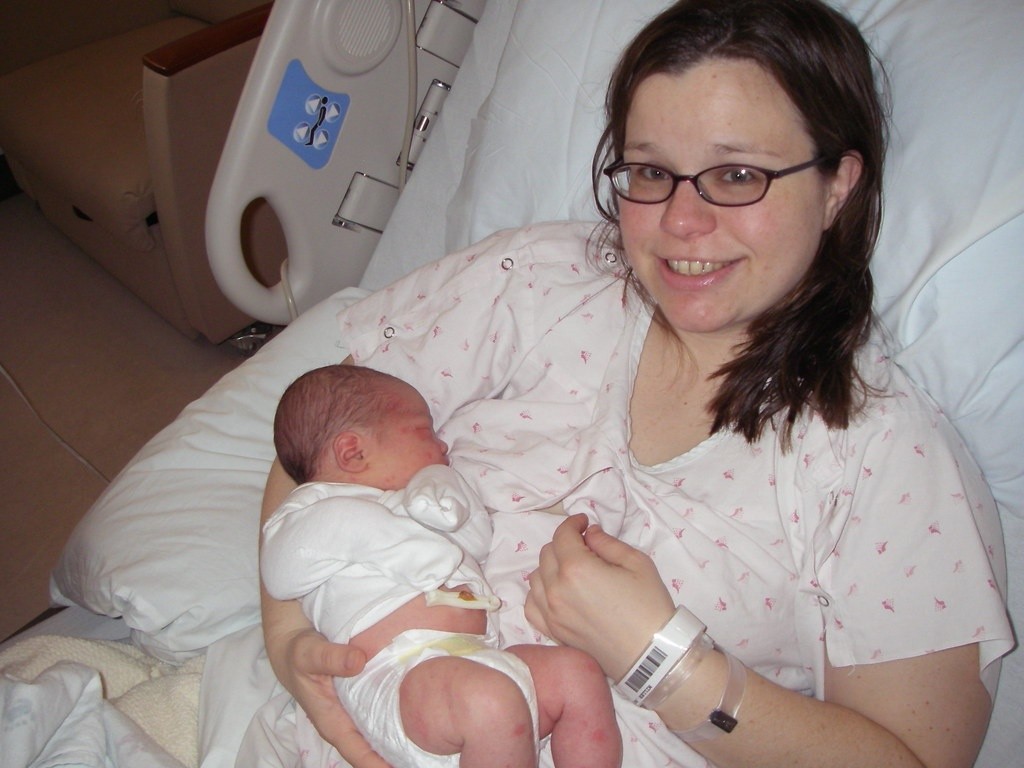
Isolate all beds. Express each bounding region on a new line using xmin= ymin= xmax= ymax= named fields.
xmin=1 ymin=0 xmax=1024 ymax=768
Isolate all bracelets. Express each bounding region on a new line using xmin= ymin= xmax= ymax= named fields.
xmin=613 ymin=606 xmax=747 ymax=743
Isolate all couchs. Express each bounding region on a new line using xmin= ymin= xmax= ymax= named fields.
xmin=0 ymin=0 xmax=288 ymax=354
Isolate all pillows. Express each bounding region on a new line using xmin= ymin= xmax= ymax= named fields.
xmin=450 ymin=0 xmax=1024 ymax=511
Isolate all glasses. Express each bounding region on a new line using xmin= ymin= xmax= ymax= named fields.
xmin=603 ymin=151 xmax=834 ymax=207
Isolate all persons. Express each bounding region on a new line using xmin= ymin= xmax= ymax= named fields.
xmin=254 ymin=2 xmax=1018 ymax=768
xmin=262 ymin=365 xmax=625 ymax=768
